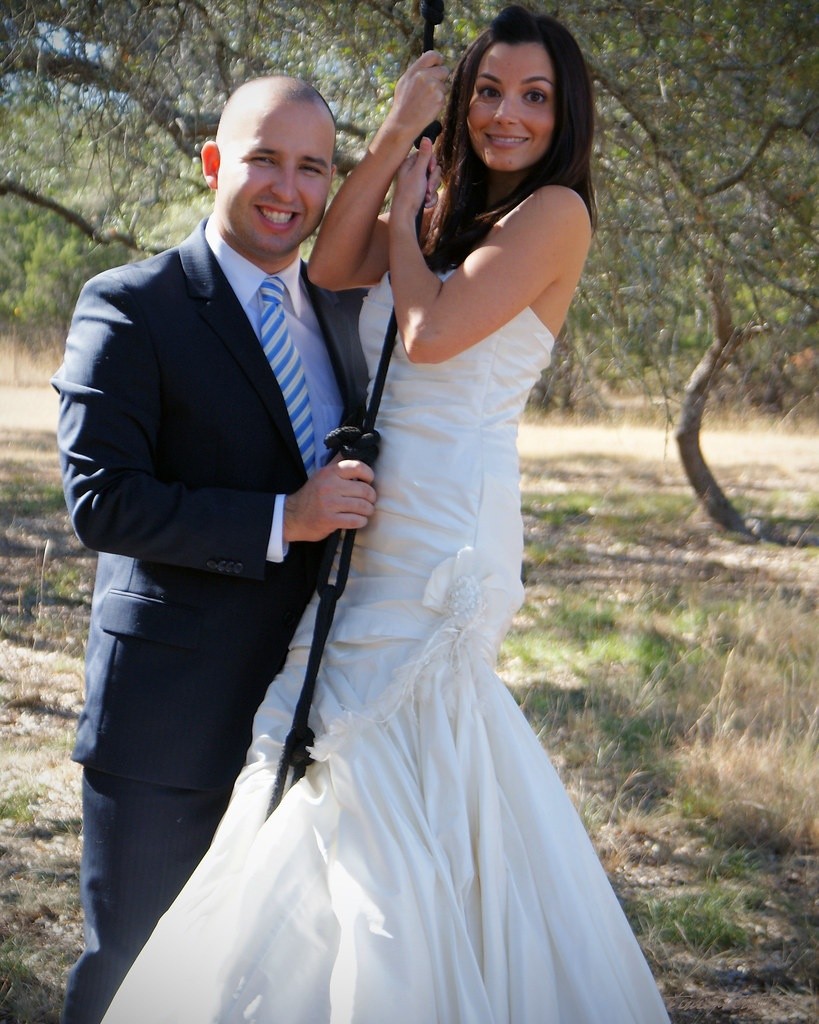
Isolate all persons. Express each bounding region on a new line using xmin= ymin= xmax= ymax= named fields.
xmin=50 ymin=77 xmax=378 ymax=1023
xmin=210 ymin=5 xmax=598 ymax=1023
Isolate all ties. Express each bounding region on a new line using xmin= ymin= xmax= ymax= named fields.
xmin=257 ymin=277 xmax=317 ymax=479
